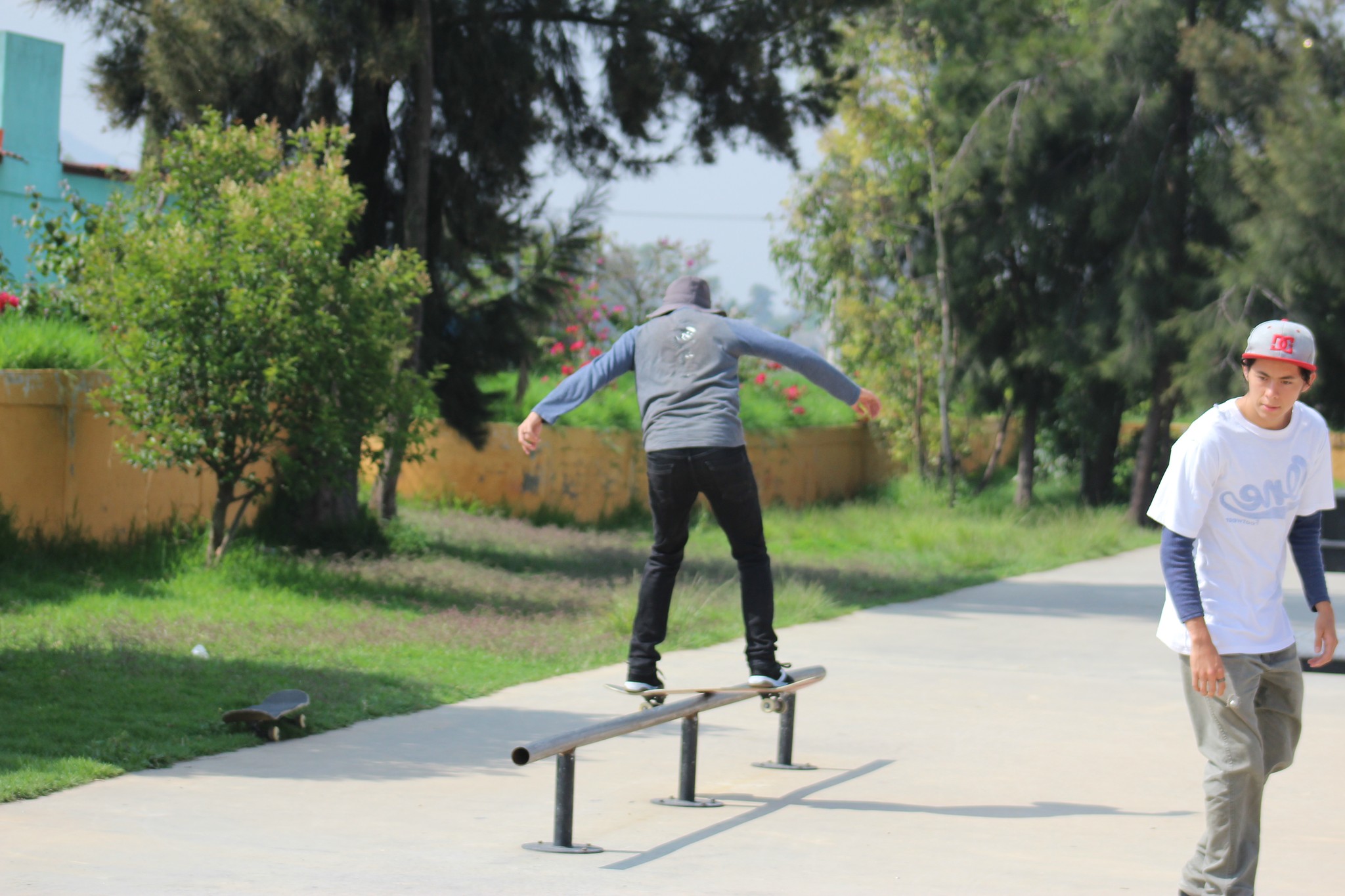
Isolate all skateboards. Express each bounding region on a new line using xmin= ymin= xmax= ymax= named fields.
xmin=605 ymin=665 xmax=828 ymax=714
xmin=222 ymin=689 xmax=312 ymax=742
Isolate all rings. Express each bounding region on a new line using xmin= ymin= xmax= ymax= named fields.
xmin=1217 ymin=677 xmax=1226 ymax=683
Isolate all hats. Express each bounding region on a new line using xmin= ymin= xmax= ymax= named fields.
xmin=647 ymin=277 xmax=725 ymax=317
xmin=1242 ymin=319 xmax=1316 ymax=370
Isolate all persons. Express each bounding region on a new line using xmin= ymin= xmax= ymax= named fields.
xmin=1147 ymin=319 xmax=1338 ymax=896
xmin=517 ymin=277 xmax=879 ymax=693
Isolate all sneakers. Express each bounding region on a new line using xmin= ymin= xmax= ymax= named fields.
xmin=625 ymin=669 xmax=666 ymax=703
xmin=749 ymin=657 xmax=794 ymax=688
